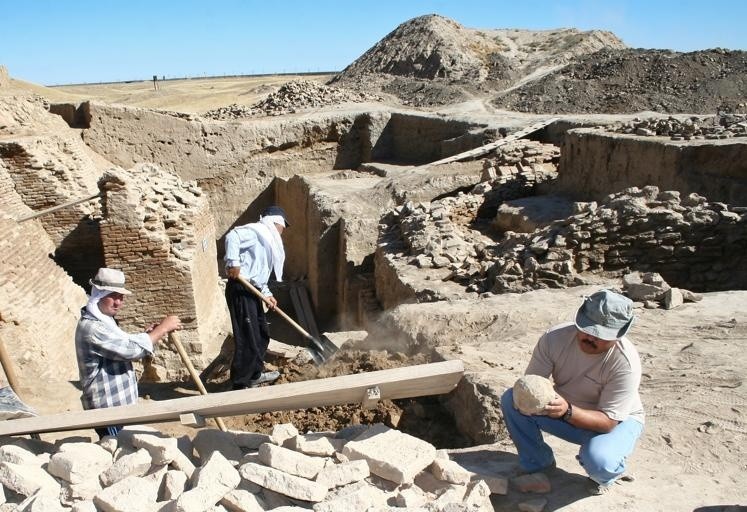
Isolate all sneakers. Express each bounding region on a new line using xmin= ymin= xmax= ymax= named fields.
xmin=251 ymin=371 xmax=279 ymax=385
xmin=0 ymin=387 xmax=39 ymax=420
xmin=587 ymin=480 xmax=611 ymax=495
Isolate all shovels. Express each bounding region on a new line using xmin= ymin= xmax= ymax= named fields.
xmin=226 ymin=265 xmax=340 ymax=365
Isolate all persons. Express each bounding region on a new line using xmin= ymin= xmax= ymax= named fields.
xmin=74 ymin=267 xmax=183 ymax=440
xmin=500 ymin=289 xmax=645 ymax=495
xmin=224 ymin=205 xmax=291 ymax=387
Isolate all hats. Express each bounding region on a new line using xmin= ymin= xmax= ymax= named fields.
xmin=575 ymin=290 xmax=635 ymax=341
xmin=89 ymin=267 xmax=133 ymax=295
xmin=257 ymin=206 xmax=291 ymax=228
